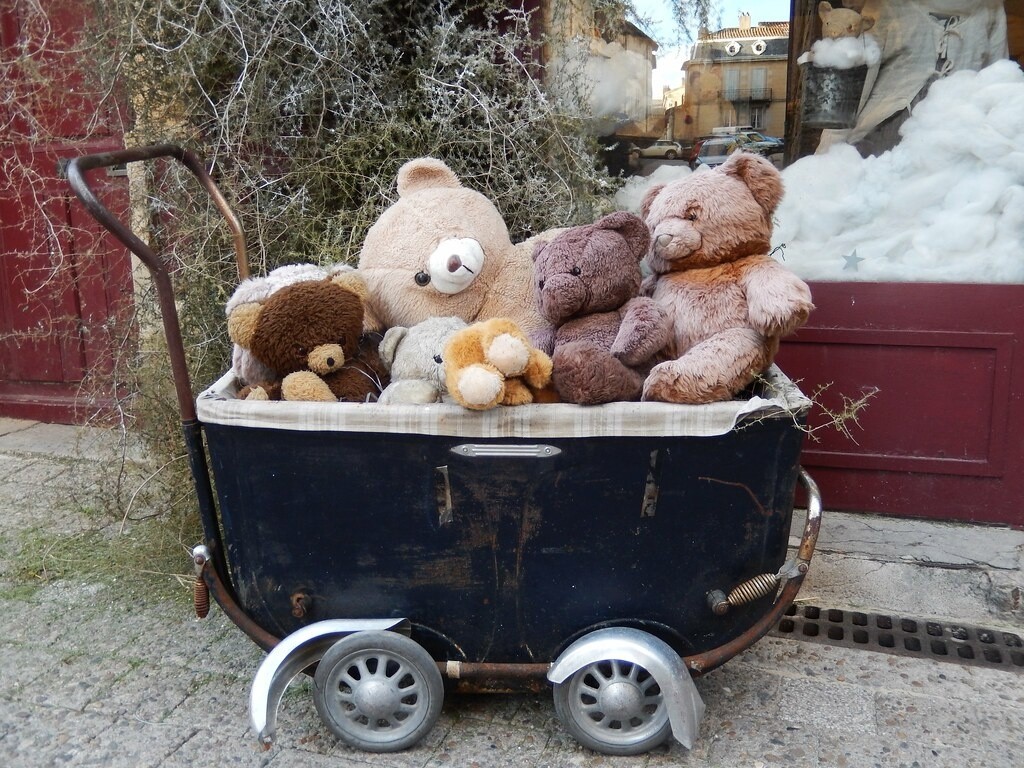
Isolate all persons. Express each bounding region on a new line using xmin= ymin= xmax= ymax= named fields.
xmin=814 ymin=1 xmax=1011 ymax=159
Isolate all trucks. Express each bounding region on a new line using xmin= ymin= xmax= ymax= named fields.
xmin=712 ymin=126 xmax=779 ymax=154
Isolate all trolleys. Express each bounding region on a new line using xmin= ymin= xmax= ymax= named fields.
xmin=69 ymin=144 xmax=823 ymax=754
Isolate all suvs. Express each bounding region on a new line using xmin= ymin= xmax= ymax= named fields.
xmin=688 ymin=140 xmax=706 ymax=171
xmin=694 ymin=138 xmax=739 ymax=170
xmin=640 ymin=140 xmax=683 ymax=159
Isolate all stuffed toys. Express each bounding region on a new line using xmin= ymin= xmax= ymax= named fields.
xmin=376 ymin=316 xmax=468 ymax=404
xmin=442 ymin=319 xmax=553 ymax=412
xmin=532 ymin=211 xmax=675 ymax=405
xmin=224 ymin=264 xmax=355 ymax=387
xmin=689 ymin=68 xmax=737 ymax=137
xmin=354 ymin=156 xmax=560 ymax=355
xmin=639 ymin=152 xmax=814 ymax=404
xmin=227 ymin=268 xmax=390 ymax=402
